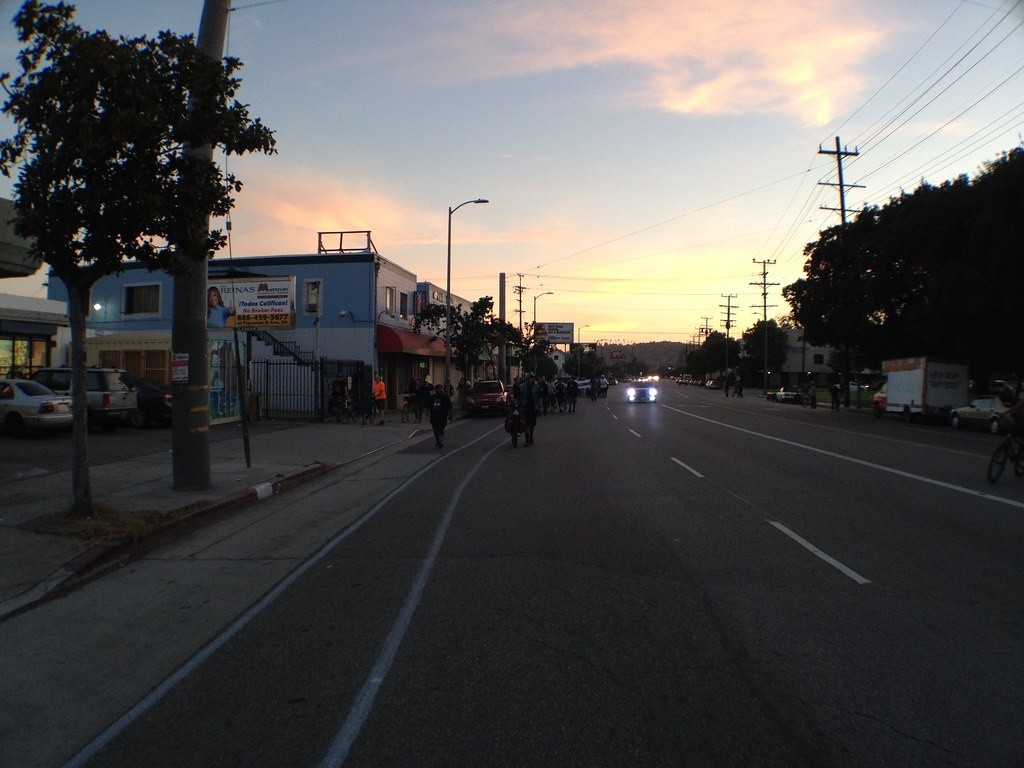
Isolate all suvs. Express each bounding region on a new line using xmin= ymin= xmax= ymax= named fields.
xmin=465 ymin=379 xmax=508 ymax=419
xmin=29 ymin=364 xmax=137 ymax=432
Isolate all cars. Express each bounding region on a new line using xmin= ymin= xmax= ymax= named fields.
xmin=128 ymin=376 xmax=174 ymax=430
xmin=949 ymin=395 xmax=1014 ymax=436
xmin=775 ymin=386 xmax=799 ymax=404
xmin=0 ymin=379 xmax=73 ymax=442
xmin=670 ymin=374 xmax=721 ymax=389
xmin=607 ymin=377 xmax=619 ymax=385
xmin=835 ymin=381 xmax=867 ymax=391
xmin=626 ymin=380 xmax=658 ymax=404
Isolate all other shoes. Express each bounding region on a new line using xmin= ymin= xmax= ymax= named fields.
xmin=336 ymin=418 xmax=342 ymax=423
xmin=542 ymin=404 xmax=576 ymax=416
xmin=436 ymin=441 xmax=443 ymax=448
xmin=415 ymin=418 xmax=422 ymax=423
xmin=380 ymin=420 xmax=384 ymax=425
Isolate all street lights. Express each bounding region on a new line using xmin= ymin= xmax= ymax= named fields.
xmin=444 ymin=197 xmax=490 ymax=398
xmin=577 ymin=325 xmax=589 ymax=379
xmin=533 ymin=291 xmax=554 ymax=375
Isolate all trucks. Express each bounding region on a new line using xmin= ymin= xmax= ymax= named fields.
xmin=872 ymin=357 xmax=968 ymax=425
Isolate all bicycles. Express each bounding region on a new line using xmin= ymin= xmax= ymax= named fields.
xmin=362 ymin=391 xmax=377 ymax=425
xmin=336 ymin=398 xmax=360 ymax=424
xmin=503 ymin=394 xmax=523 ymax=450
xmin=400 ymin=393 xmax=417 ymax=423
xmin=986 ymin=420 xmax=1024 ymax=484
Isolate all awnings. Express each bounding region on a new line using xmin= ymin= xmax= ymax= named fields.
xmin=376 ymin=324 xmax=446 ymax=357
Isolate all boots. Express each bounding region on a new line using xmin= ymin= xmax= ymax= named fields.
xmin=524 ymin=433 xmax=530 ymax=447
xmin=529 ymin=431 xmax=534 ymax=444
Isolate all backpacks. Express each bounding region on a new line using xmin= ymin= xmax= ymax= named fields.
xmin=449 ymin=385 xmax=455 ymax=396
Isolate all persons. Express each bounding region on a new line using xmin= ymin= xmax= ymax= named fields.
xmin=724 ymin=380 xmax=743 ymax=398
xmin=809 ymin=384 xmax=817 ymax=409
xmin=534 ymin=375 xmax=578 ymax=416
xmin=515 ymin=372 xmax=541 ymax=447
xmin=829 ymin=384 xmax=840 ymax=412
xmin=1006 ymin=376 xmax=1024 ymax=439
xmin=508 ymin=377 xmax=521 ymax=401
xmin=373 ymin=374 xmax=386 ymax=426
xmin=591 ymin=376 xmax=597 ymax=401
xmin=447 ymin=378 xmax=454 ymax=396
xmin=427 ymin=384 xmax=450 ymax=448
xmin=599 ymin=375 xmax=608 ymax=398
xmin=331 ymin=372 xmax=348 ymax=423
xmin=207 ymin=286 xmax=234 ymax=328
xmin=408 ymin=376 xmax=435 ymax=423
xmin=457 ymin=377 xmax=471 ymax=393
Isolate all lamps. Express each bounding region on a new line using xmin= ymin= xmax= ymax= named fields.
xmin=378 ymin=310 xmax=397 ymax=321
xmin=340 ymin=310 xmax=352 ymax=321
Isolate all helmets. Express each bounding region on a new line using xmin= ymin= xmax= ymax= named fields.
xmin=601 ymin=374 xmax=605 ymax=378
xmin=525 ymin=372 xmax=535 ymax=380
